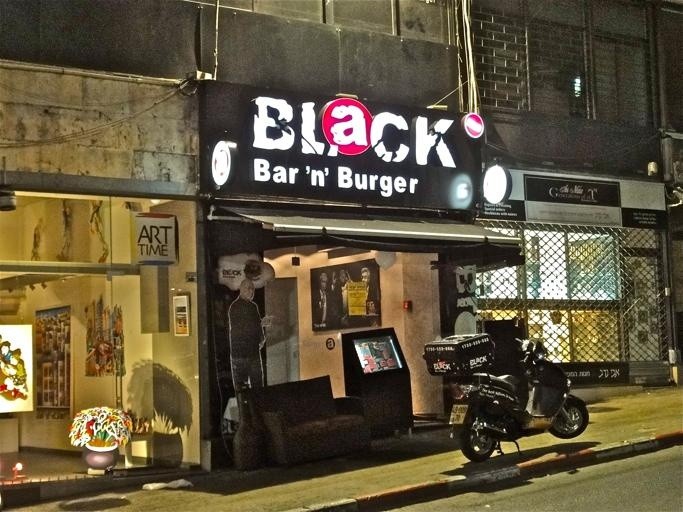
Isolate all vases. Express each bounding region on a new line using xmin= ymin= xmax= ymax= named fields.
xmin=85 ymin=443 xmax=118 ymax=470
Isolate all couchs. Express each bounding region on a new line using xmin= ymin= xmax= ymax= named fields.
xmin=231 ymin=375 xmax=384 ymax=470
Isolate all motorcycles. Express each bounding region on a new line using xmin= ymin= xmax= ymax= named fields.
xmin=421 ymin=334 xmax=588 ymax=464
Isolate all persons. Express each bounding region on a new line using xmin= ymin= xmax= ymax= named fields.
xmin=228 ymin=281 xmax=266 ymax=393
xmin=314 ymin=267 xmax=377 ymax=329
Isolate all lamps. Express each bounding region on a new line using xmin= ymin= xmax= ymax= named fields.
xmin=11 ymin=463 xmax=23 ymax=478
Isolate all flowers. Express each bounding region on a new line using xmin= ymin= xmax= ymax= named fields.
xmin=69 ymin=407 xmax=133 ymax=448
